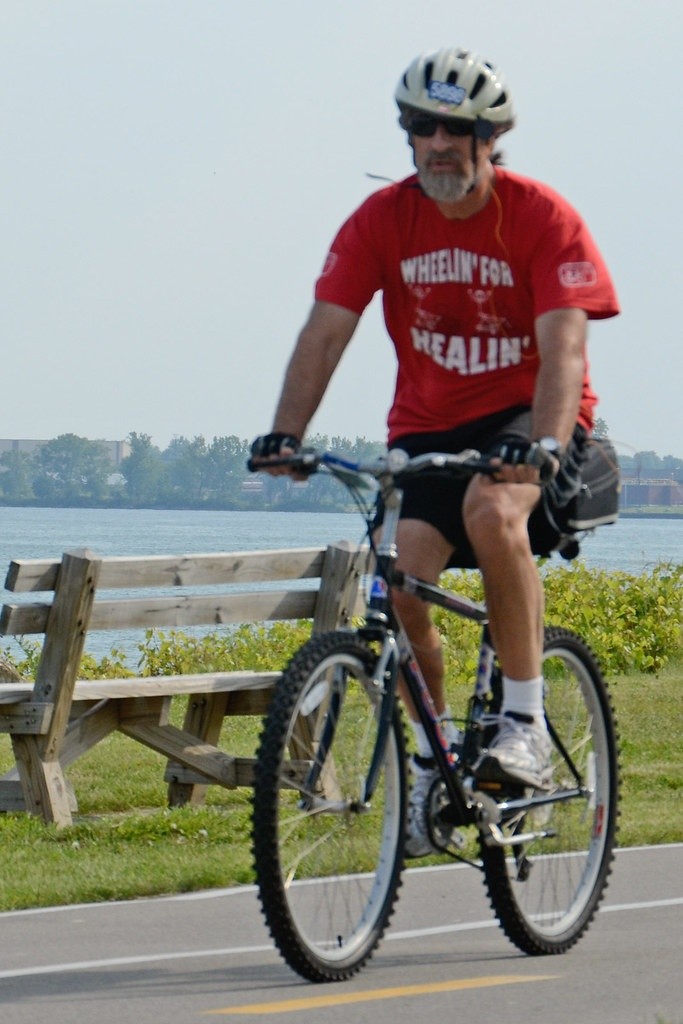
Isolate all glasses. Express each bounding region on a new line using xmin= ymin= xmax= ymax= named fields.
xmin=400 ymin=114 xmax=474 ymax=138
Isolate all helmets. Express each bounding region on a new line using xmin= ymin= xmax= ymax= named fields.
xmin=395 ymin=44 xmax=515 ymax=124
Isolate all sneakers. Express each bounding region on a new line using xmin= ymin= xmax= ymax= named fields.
xmin=401 ymin=730 xmax=463 ymax=860
xmin=472 ymin=711 xmax=555 ymax=797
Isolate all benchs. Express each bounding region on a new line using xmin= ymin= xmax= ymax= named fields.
xmin=0 ymin=539 xmax=375 ymax=832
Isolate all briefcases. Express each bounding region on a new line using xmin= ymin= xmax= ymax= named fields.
xmin=534 ymin=438 xmax=621 ymax=535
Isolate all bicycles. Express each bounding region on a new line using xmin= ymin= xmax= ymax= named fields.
xmin=248 ymin=443 xmax=624 ymax=985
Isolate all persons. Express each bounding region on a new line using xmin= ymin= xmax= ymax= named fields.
xmin=247 ymin=46 xmax=619 ymax=859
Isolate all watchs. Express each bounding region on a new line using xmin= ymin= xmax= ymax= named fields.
xmin=534 ymin=436 xmax=561 ymax=456
